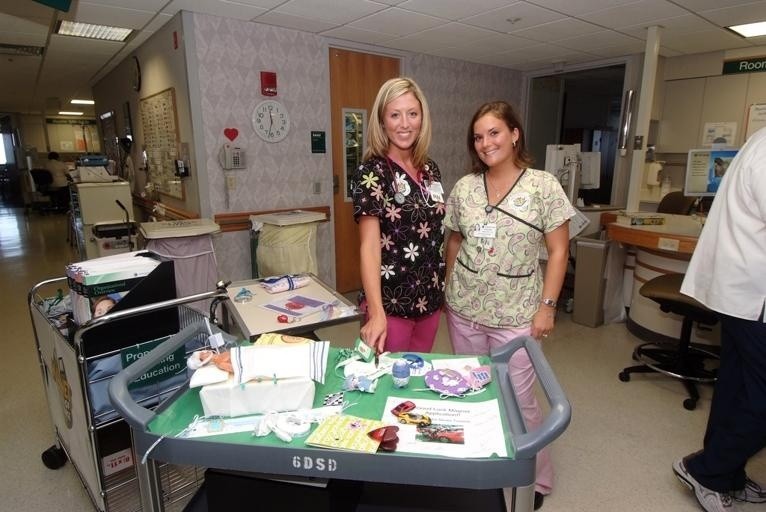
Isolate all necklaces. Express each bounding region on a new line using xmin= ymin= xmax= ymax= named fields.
xmin=487 ymin=174 xmax=514 ymax=198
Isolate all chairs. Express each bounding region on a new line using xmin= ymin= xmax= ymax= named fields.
xmin=657 ymin=190 xmax=698 ymax=215
xmin=30 ymin=168 xmax=66 ymax=216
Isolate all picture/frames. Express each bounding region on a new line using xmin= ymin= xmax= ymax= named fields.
xmin=139 ymin=87 xmax=186 ymax=201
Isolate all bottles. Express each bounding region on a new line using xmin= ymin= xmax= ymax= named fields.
xmin=660 ymin=177 xmax=671 ymax=200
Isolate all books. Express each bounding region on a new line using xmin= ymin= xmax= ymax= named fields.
xmin=617 ymin=209 xmax=665 ymax=226
xmin=65 ymin=249 xmax=162 ymax=327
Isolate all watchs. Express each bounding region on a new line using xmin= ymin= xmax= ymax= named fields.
xmin=539 ymin=298 xmax=557 ymax=308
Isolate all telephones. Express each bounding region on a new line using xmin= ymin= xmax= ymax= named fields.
xmin=219 ymin=143 xmax=247 ymax=169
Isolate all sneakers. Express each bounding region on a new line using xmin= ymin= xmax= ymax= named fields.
xmin=729 ymin=475 xmax=766 ymax=504
xmin=534 ymin=491 xmax=545 ymax=509
xmin=672 ymin=452 xmax=736 ymax=512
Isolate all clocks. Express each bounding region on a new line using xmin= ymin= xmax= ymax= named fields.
xmin=252 ymin=99 xmax=290 ymax=143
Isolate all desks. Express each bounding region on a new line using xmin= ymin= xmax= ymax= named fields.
xmin=69 ymin=177 xmax=134 ymax=260
xmin=602 ymin=211 xmax=723 ymax=360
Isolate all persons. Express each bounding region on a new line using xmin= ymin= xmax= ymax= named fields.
xmin=352 ymin=77 xmax=449 ymax=355
xmin=443 ymin=101 xmax=577 ymax=510
xmin=44 ymin=151 xmax=74 ymax=215
xmin=672 ymin=123 xmax=766 ymax=512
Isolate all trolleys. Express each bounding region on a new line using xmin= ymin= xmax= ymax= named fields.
xmin=116 ymin=318 xmax=573 ymax=509
xmin=25 ymin=273 xmax=216 ymax=507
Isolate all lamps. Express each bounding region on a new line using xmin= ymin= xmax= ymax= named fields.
xmin=71 ymin=99 xmax=95 ymax=105
xmin=58 ymin=111 xmax=85 ymax=116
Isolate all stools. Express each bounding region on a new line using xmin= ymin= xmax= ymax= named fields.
xmin=618 ymin=273 xmax=722 ymax=410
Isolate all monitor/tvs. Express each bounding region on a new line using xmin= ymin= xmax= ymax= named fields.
xmin=544 ymin=143 xmax=602 ymax=206
xmin=684 ymin=148 xmax=741 ymax=197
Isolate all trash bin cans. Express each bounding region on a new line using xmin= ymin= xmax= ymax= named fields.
xmin=573 ymin=231 xmax=613 ymax=328
